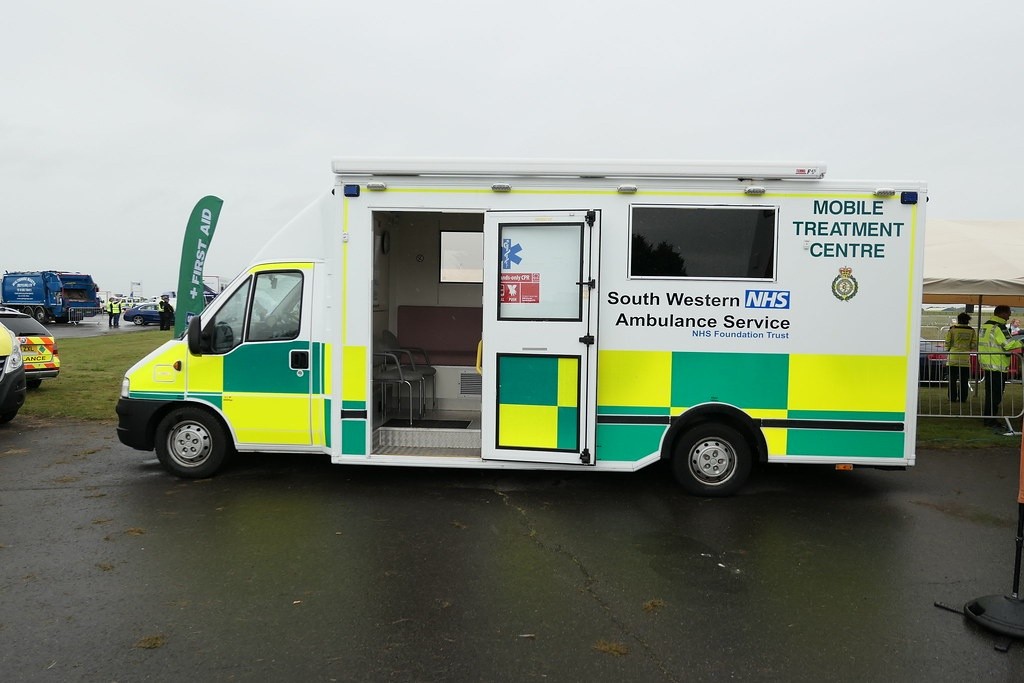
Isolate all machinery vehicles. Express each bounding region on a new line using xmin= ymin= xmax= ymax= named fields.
xmin=0 ymin=270 xmax=104 ymax=325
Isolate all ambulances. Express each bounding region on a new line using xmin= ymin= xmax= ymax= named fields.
xmin=0 ymin=307 xmax=60 ymax=389
xmin=115 ymin=160 xmax=938 ymax=498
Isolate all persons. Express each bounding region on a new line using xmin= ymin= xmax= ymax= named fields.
xmin=1006 ymin=318 xmax=1020 ymax=336
xmin=107 ymin=297 xmax=121 ymax=327
xmin=944 ymin=312 xmax=977 ymax=404
xmin=978 ymin=305 xmax=1024 ymax=428
xmin=158 ymin=295 xmax=174 ymax=331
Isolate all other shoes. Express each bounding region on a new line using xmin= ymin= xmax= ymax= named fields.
xmin=988 ymin=421 xmax=1007 ymax=429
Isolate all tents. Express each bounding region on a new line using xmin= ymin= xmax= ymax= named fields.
xmin=203 ymin=283 xmax=219 ymax=306
xmin=923 ymin=220 xmax=1024 ymax=306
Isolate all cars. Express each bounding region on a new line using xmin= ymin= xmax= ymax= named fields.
xmin=120 ymin=296 xmax=148 ymax=310
xmin=0 ymin=322 xmax=26 ymax=428
xmin=123 ymin=303 xmax=175 ymax=325
xmin=920 ymin=337 xmax=950 ymax=386
xmin=155 ymin=291 xmax=177 ymax=312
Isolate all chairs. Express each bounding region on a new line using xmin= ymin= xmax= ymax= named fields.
xmin=372 ymin=333 xmax=423 ymax=425
xmin=382 ymin=330 xmax=438 ymax=417
